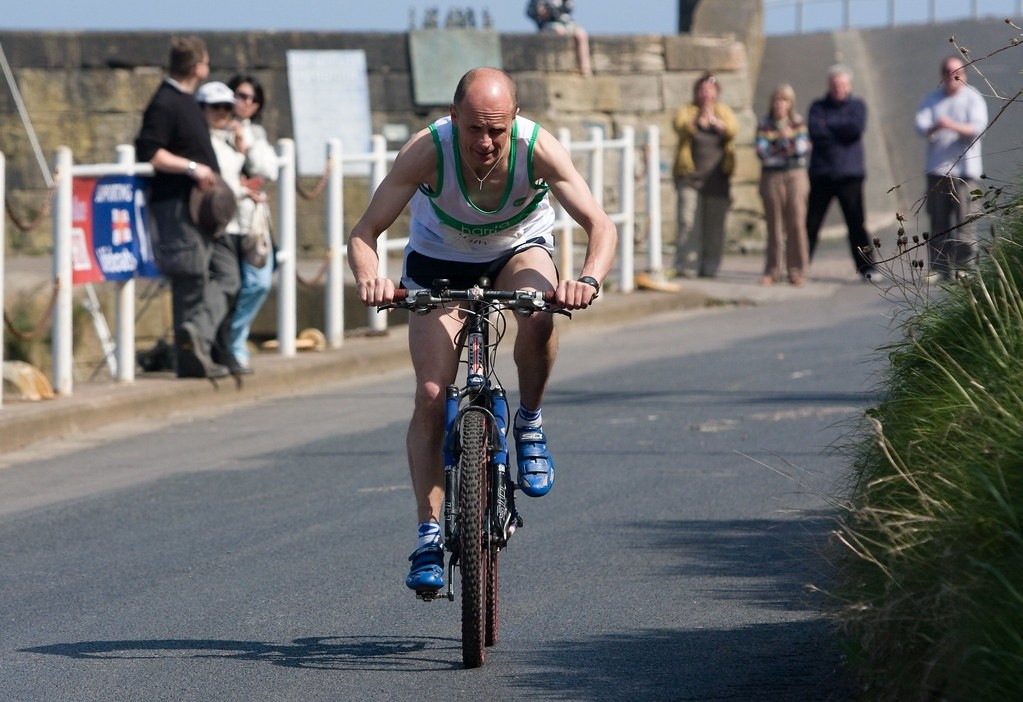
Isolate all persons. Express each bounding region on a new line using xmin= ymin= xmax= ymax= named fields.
xmin=671 ymin=70 xmax=737 ymax=279
xmin=806 ymin=63 xmax=886 ymax=282
xmin=133 ymin=37 xmax=279 ymax=381
xmin=346 ymin=68 xmax=618 ymax=592
xmin=755 ymin=83 xmax=813 ymax=287
xmin=527 ymin=0 xmax=594 ymax=78
xmin=913 ymin=55 xmax=988 ymax=285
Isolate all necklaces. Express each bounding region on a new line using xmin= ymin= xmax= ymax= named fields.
xmin=458 ymin=142 xmax=508 ymax=190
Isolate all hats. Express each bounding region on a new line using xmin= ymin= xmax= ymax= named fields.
xmin=190 ymin=174 xmax=237 ymax=238
xmin=196 ymin=81 xmax=237 ymax=104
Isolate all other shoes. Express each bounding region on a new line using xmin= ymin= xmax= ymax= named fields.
xmin=232 ymin=362 xmax=253 ymax=377
xmin=174 ymin=325 xmax=229 ymax=378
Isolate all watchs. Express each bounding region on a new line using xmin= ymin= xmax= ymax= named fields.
xmin=576 ymin=276 xmax=602 ymax=292
xmin=187 ymin=160 xmax=196 ymax=177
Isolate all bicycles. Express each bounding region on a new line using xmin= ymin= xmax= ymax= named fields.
xmin=365 ymin=283 xmax=598 ymax=669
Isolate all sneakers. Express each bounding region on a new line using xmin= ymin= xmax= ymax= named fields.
xmin=405 ymin=544 xmax=445 ymax=589
xmin=513 ymin=410 xmax=554 ymax=497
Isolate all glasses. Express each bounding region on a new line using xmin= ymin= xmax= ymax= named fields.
xmin=202 ymin=103 xmax=233 ymax=112
xmin=236 ymin=92 xmax=259 ymax=103
